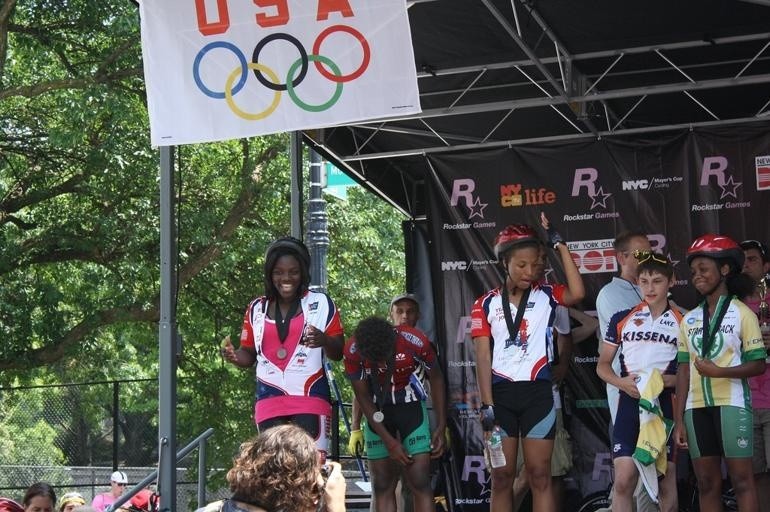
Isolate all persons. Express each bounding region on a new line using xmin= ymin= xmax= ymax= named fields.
xmin=1 ymin=472 xmax=158 ymax=512
xmin=192 ymin=423 xmax=346 ymax=512
xmin=339 ymin=293 xmax=449 ymax=512
xmin=470 ymin=212 xmax=770 ymax=512
xmin=222 ymin=237 xmax=344 ymax=464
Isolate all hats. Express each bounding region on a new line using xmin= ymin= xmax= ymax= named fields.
xmin=0 ymin=497 xmax=26 ymax=511
xmin=59 ymin=492 xmax=86 ymax=511
xmin=391 ymin=292 xmax=419 ymax=310
xmin=111 ymin=471 xmax=128 ymax=486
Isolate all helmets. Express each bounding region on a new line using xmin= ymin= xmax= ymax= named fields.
xmin=683 ymin=232 xmax=746 ymax=273
xmin=265 ymin=236 xmax=310 ymax=285
xmin=490 ymin=224 xmax=545 ymax=263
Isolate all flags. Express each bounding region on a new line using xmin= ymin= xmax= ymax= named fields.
xmin=138 ymin=0 xmax=423 ymax=148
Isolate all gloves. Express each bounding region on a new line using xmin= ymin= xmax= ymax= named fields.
xmin=346 ymin=427 xmax=365 ymax=457
xmin=477 ymin=402 xmax=499 ymax=434
xmin=545 ymin=220 xmax=568 ymax=253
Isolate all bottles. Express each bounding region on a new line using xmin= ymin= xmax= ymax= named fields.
xmin=485 ymin=424 xmax=507 ymax=469
